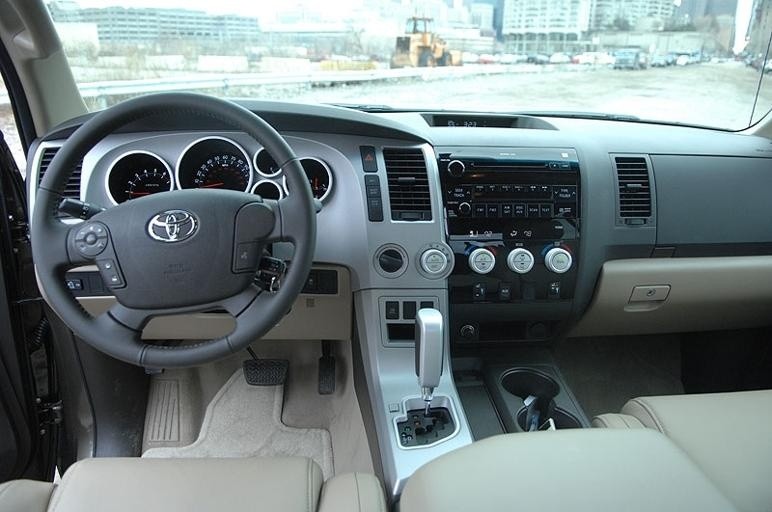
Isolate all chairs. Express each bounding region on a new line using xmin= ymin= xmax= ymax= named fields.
xmin=0 ymin=455 xmax=386 ymax=512
xmin=591 ymin=388 xmax=771 ymax=512
